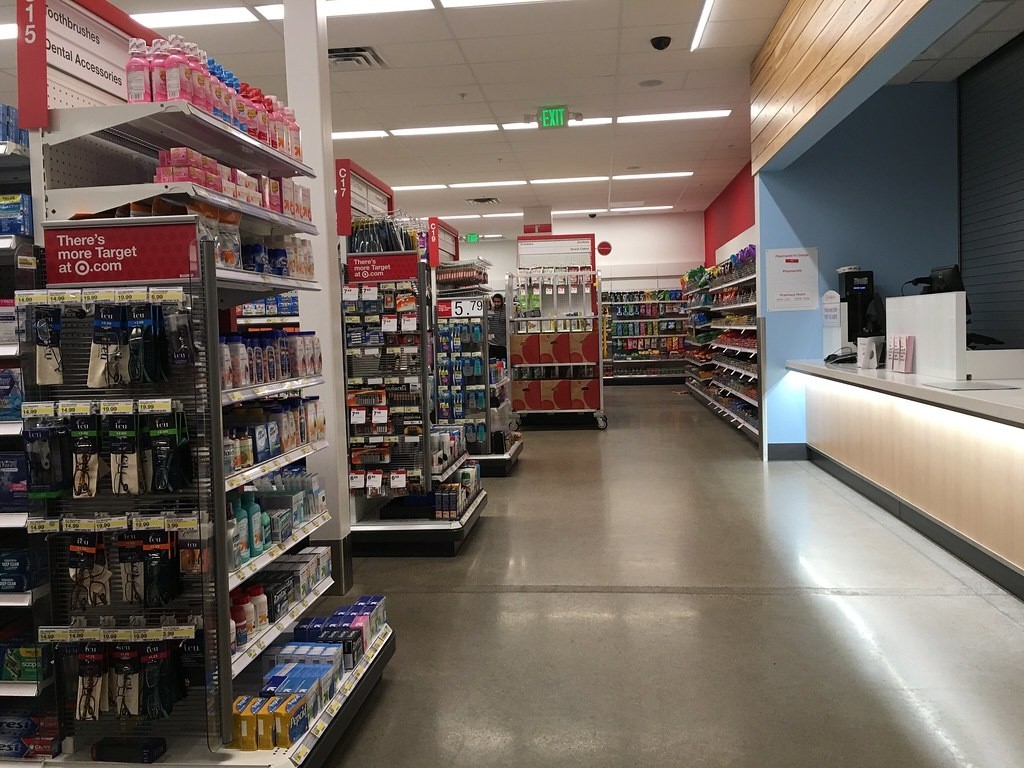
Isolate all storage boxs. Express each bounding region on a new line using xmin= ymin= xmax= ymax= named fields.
xmin=508 ymin=332 xmax=600 ymax=410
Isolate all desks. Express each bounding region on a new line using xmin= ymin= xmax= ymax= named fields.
xmin=785 ymin=359 xmax=1024 ymax=601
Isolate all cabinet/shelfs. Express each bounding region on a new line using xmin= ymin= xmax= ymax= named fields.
xmin=602 ymin=285 xmax=688 ymax=383
xmin=0 ymin=123 xmax=49 ymax=765
xmin=505 ymin=326 xmax=602 ymax=414
xmin=709 ymin=274 xmax=759 ymax=438
xmin=348 ymin=449 xmax=488 ymax=557
xmin=17 ymin=0 xmax=396 ymax=768
xmin=467 ymin=376 xmax=523 ymax=473
xmin=683 ymin=285 xmax=710 ymax=397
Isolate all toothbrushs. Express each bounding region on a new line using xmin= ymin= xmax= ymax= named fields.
xmin=437 ymin=324 xmax=463 ymax=418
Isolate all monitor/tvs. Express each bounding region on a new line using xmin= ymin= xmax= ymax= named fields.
xmin=866 ymin=293 xmax=886 ymax=331
xmin=931 ymin=264 xmax=972 ymax=315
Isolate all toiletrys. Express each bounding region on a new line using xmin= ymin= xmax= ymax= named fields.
xmin=229 ymin=584 xmax=269 ymax=657
xmin=124 ymin=34 xmax=304 ymax=163
xmin=226 ymin=487 xmax=272 ymax=573
xmin=218 ymin=329 xmax=323 ymax=390
xmin=263 ymin=234 xmax=315 ymax=280
xmin=223 ymin=395 xmax=327 ymax=477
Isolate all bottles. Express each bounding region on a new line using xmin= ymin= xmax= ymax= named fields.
xmin=226 ymin=493 xmax=263 ymax=571
xmin=223 ymin=397 xmax=326 ymax=475
xmin=230 ymin=586 xmax=268 ymax=658
xmin=220 ymin=331 xmax=322 ymax=389
xmin=127 ymin=35 xmax=304 ymax=162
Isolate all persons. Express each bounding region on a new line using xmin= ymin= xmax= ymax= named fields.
xmin=488 ymin=294 xmax=507 ymax=360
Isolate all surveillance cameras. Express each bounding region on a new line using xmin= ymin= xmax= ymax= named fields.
xmin=589 ymin=214 xmax=597 ymax=218
xmin=650 ymin=36 xmax=670 ymax=50
